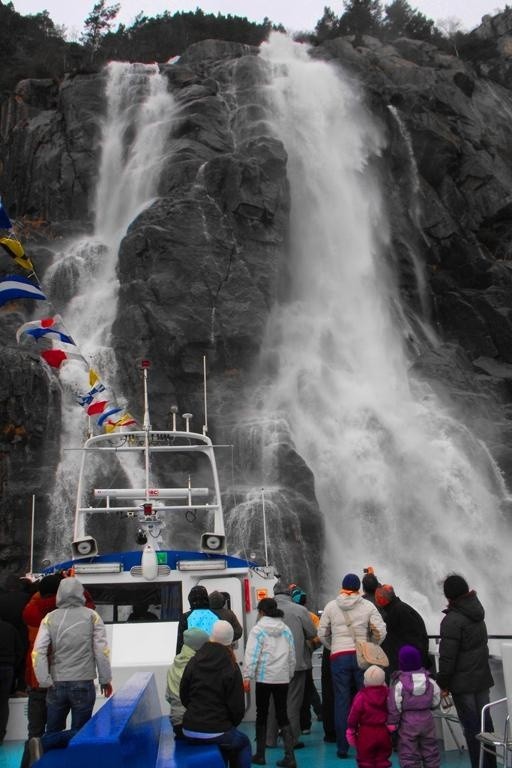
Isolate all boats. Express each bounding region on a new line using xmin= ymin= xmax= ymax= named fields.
xmin=0 ymin=356 xmax=510 ymax=767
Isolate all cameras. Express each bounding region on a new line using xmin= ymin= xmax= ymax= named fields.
xmin=55 ymin=569 xmax=69 ymax=576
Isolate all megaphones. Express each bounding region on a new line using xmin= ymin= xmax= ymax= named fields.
xmin=200 ymin=533 xmax=227 ymax=554
xmin=72 ymin=535 xmax=99 ymax=560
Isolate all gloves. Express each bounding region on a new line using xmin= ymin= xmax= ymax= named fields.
xmin=345 ymin=728 xmax=357 ymax=748
xmin=243 ymin=679 xmax=250 ymax=692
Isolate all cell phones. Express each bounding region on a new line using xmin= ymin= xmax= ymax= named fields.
xmin=364 ymin=569 xmax=368 ymax=573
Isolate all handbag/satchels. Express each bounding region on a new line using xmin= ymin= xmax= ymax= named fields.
xmin=355 ymin=639 xmax=389 ymax=669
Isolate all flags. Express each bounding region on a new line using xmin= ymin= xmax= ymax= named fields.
xmin=0 ymin=189 xmax=139 ymax=434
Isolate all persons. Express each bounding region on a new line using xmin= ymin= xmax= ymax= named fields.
xmin=23 ymin=574 xmax=95 ymax=736
xmin=317 ymin=574 xmax=386 ymax=759
xmin=0 ymin=620 xmax=25 ymax=743
xmin=179 ymin=620 xmax=252 ymax=767
xmin=31 ymin=577 xmax=112 ymax=731
xmin=346 ymin=665 xmax=392 ymax=767
xmin=387 ymin=645 xmax=442 ymax=767
xmin=0 ymin=576 xmax=29 ymax=698
xmin=257 ymin=580 xmax=322 ymax=750
xmin=322 ymin=574 xmax=429 ymax=743
xmin=243 ymin=598 xmax=297 ymax=767
xmin=435 ymin=575 xmax=494 ymax=767
xmin=168 ymin=585 xmax=243 ymax=736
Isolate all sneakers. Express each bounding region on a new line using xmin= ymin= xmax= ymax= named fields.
xmin=28 ymin=737 xmax=43 ymax=767
xmin=251 ymin=712 xmax=321 ymax=767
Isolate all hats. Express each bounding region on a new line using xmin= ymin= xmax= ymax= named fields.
xmin=258 ymin=579 xmax=306 ymax=611
xmin=362 ymin=663 xmax=385 ymax=686
xmin=342 ymin=573 xmax=396 ymax=606
xmin=444 ymin=576 xmax=468 ymax=599
xmin=183 ymin=620 xmax=235 ymax=651
xmin=37 ymin=575 xmax=60 ymax=598
xmin=396 ymin=646 xmax=422 ymax=670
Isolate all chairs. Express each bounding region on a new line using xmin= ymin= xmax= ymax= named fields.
xmin=474 ymin=696 xmax=512 ymax=768
